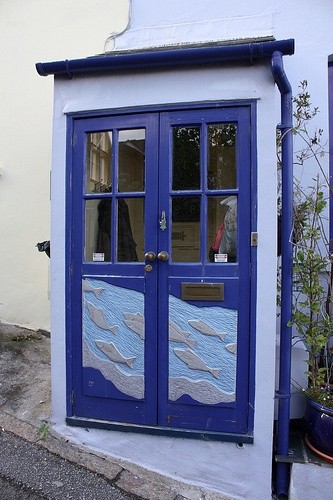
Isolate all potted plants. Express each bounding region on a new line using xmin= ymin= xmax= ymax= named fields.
xmin=274 ymin=78 xmax=333 ymax=463
